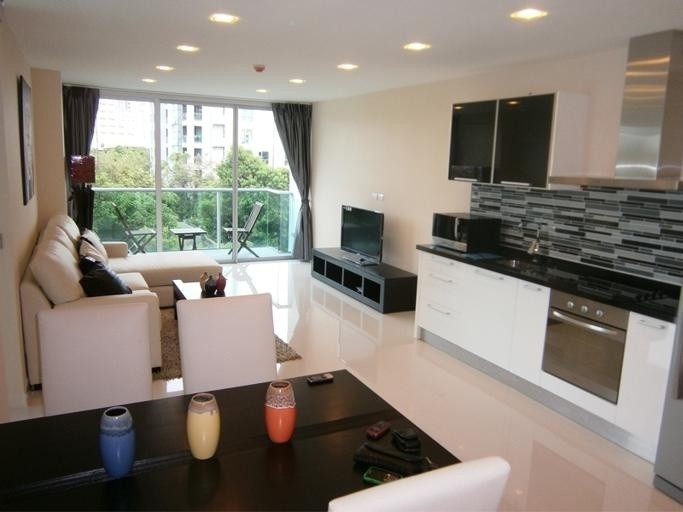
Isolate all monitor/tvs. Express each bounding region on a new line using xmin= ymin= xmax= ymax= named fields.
xmin=448 ymin=99 xmax=498 ymax=185
xmin=491 ymin=93 xmax=559 ymax=189
xmin=339 ymin=205 xmax=384 ymax=266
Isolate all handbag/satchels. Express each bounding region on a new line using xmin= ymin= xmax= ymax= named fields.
xmin=353 ymin=440 xmax=429 ymax=477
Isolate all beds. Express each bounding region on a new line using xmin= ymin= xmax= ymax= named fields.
xmin=33 ymin=214 xmax=223 ymax=308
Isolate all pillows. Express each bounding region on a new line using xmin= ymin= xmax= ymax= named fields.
xmin=79 ymin=228 xmax=132 ymax=297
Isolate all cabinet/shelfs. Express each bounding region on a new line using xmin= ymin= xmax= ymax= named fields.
xmin=413 ymin=250 xmax=518 ymax=385
xmin=310 ymin=247 xmax=417 ymax=314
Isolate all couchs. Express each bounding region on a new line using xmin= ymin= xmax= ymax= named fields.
xmin=19 ymin=226 xmax=162 ymax=392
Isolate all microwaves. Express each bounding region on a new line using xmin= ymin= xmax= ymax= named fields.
xmin=431 ymin=212 xmax=502 ymax=252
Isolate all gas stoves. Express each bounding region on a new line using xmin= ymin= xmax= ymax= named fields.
xmin=550 ymin=268 xmax=670 ymax=331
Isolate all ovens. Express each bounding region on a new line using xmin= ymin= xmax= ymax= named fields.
xmin=541 ymin=306 xmax=627 ymax=406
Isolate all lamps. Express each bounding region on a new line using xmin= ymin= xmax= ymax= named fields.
xmin=70 ymin=154 xmax=95 ymax=228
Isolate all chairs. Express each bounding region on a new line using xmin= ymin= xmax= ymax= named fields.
xmin=327 ymin=456 xmax=511 ymax=512
xmin=224 ymin=201 xmax=265 ymax=258
xmin=176 ymin=293 xmax=278 ymax=395
xmin=112 ymin=202 xmax=157 ymax=255
xmin=36 ymin=302 xmax=152 ymax=417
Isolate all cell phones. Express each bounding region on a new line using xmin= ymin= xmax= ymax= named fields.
xmin=367 ymin=420 xmax=390 ymax=440
xmin=363 ymin=465 xmax=403 ymax=485
xmin=307 ymin=373 xmax=334 ymax=384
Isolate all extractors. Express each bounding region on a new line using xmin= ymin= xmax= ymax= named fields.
xmin=547 ymin=28 xmax=683 ymax=199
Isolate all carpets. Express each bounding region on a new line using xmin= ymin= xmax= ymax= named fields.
xmin=152 ymin=305 xmax=302 ymax=380
